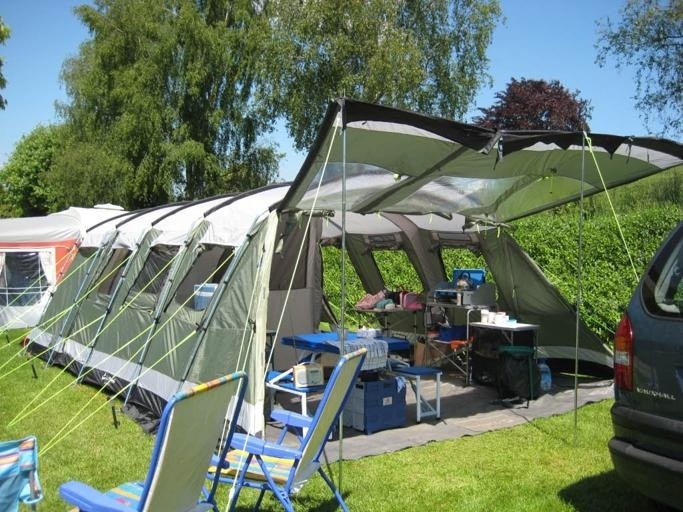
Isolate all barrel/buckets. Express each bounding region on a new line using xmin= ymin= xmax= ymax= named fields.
xmin=538 ymin=357 xmax=554 ymax=391
xmin=193 ymin=284 xmax=217 ymax=310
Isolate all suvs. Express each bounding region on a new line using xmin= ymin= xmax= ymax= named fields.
xmin=606 ymin=219 xmax=683 ymax=510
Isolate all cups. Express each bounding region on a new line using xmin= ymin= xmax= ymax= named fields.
xmin=480 ymin=309 xmax=509 ymax=325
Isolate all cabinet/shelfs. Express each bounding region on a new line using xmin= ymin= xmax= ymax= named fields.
xmin=350 ymin=283 xmax=541 ymax=400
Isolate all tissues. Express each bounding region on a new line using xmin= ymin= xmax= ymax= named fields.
xmin=357 ymin=324 xmax=382 ymax=338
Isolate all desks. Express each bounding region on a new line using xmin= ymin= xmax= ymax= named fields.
xmin=280 ymin=331 xmax=409 ymax=429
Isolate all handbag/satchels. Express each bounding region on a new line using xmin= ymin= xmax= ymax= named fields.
xmin=470 ymin=330 xmax=540 ymax=401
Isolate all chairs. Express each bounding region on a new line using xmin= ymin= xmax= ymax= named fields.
xmin=198 ymin=347 xmax=368 ymax=511
xmin=58 ymin=370 xmax=249 ymax=512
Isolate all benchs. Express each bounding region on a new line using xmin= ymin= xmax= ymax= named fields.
xmin=262 ymin=365 xmax=444 ymax=440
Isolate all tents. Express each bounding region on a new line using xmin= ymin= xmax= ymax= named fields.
xmin=0 ymin=215 xmax=82 ymax=331
xmin=47 ymin=200 xmax=130 ymax=231
xmin=19 ymin=94 xmax=683 ymax=475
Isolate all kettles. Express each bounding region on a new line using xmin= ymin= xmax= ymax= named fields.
xmin=455 ymin=271 xmax=475 ymax=291
